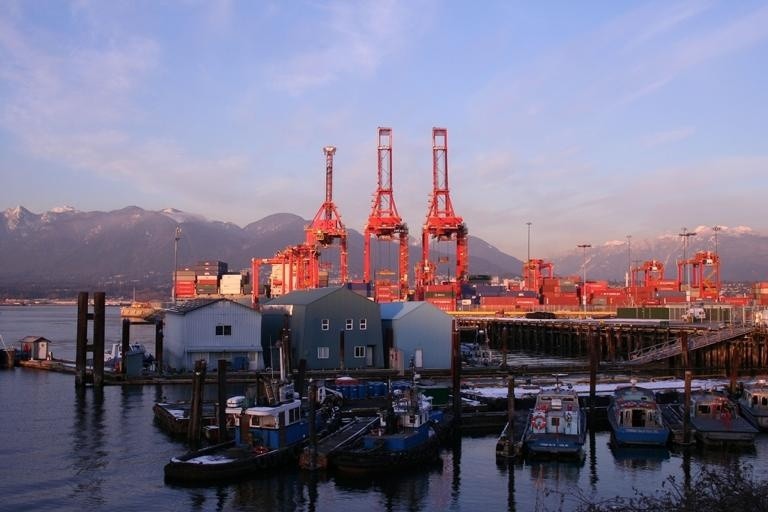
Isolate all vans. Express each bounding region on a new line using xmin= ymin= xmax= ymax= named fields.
xmin=680 ymin=308 xmax=706 ymax=323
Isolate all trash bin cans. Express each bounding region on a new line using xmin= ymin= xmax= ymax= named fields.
xmin=125 ymin=350 xmax=145 ymax=377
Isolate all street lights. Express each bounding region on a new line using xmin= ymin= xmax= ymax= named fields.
xmin=577 ymin=244 xmax=593 ymax=312
xmin=525 ymin=222 xmax=533 ymax=288
xmin=172 ymin=226 xmax=183 ymax=302
xmin=626 ymin=235 xmax=632 ymax=287
xmin=712 ymin=226 xmax=722 ymax=257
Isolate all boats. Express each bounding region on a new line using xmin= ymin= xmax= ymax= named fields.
xmin=739 ymin=388 xmax=768 ymax=431
xmin=166 ymin=378 xmax=457 ymax=486
xmin=676 ymin=391 xmax=760 ymax=443
xmin=495 ymin=411 xmax=526 ymax=460
xmin=607 ymin=379 xmax=671 ymax=444
xmin=655 ymin=400 xmax=697 ymax=445
xmin=84 ymin=342 xmax=153 ymax=371
xmin=120 ymin=284 xmax=160 ymax=324
xmin=524 ymin=388 xmax=587 ymax=453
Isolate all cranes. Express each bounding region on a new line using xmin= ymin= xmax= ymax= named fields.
xmin=250 ymin=122 xmax=767 ymax=324
